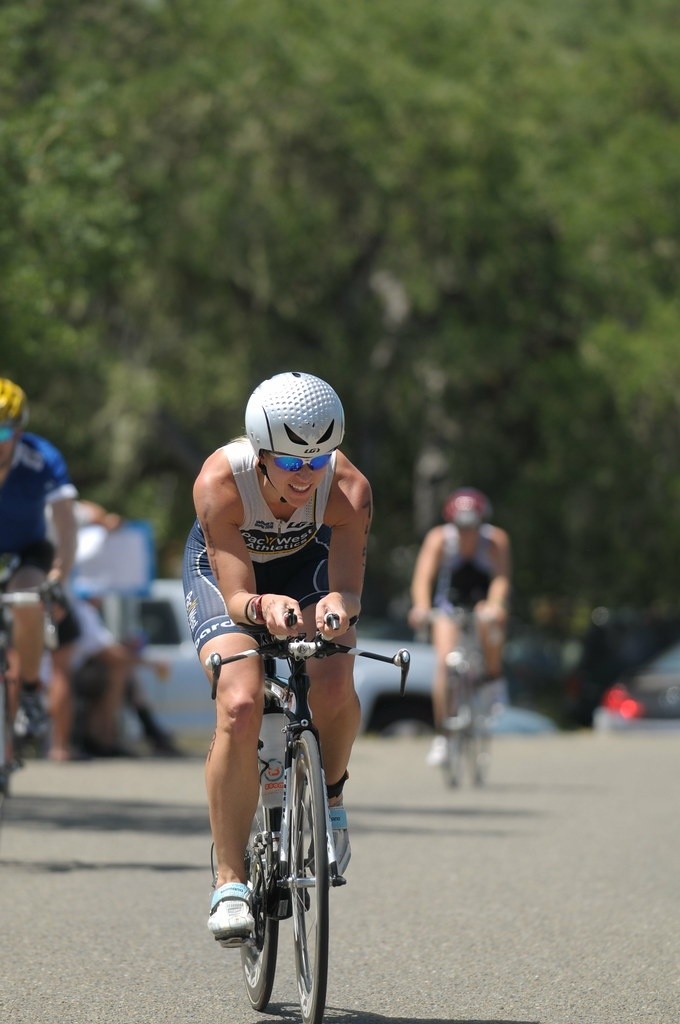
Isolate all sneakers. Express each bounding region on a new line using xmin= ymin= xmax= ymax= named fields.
xmin=307 ymin=796 xmax=351 ymax=882
xmin=207 ymin=882 xmax=257 ymax=937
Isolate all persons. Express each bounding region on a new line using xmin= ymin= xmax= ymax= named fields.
xmin=408 ymin=487 xmax=511 ymax=766
xmin=0 ymin=379 xmax=187 ymax=793
xmin=185 ymin=370 xmax=373 ymax=949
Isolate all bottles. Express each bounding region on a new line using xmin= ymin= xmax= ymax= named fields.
xmin=258 ymin=697 xmax=288 ymax=807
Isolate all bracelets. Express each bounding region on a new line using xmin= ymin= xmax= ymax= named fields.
xmin=244 ymin=596 xmax=261 ymax=626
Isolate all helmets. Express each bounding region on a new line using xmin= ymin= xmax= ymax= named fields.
xmin=443 ymin=490 xmax=490 ymax=527
xmin=1 ymin=378 xmax=26 ymax=421
xmin=245 ymin=371 xmax=344 ymax=462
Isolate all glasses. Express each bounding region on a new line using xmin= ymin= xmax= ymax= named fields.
xmin=269 ymin=452 xmax=333 ymax=471
xmin=0 ymin=427 xmax=16 ymax=440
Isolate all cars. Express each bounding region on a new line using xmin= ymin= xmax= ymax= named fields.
xmin=124 ymin=574 xmax=442 ymax=755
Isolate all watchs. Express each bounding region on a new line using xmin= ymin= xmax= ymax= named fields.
xmin=251 ymin=594 xmax=266 ymax=624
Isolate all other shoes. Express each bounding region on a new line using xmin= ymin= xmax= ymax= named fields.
xmin=483 ymin=680 xmax=505 ymax=721
xmin=427 ymin=734 xmax=447 ymax=767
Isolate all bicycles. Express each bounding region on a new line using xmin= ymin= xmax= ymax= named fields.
xmin=203 ymin=608 xmax=417 ymax=1024
xmin=411 ymin=605 xmax=509 ymax=792
xmin=0 ymin=570 xmax=63 ymax=799
